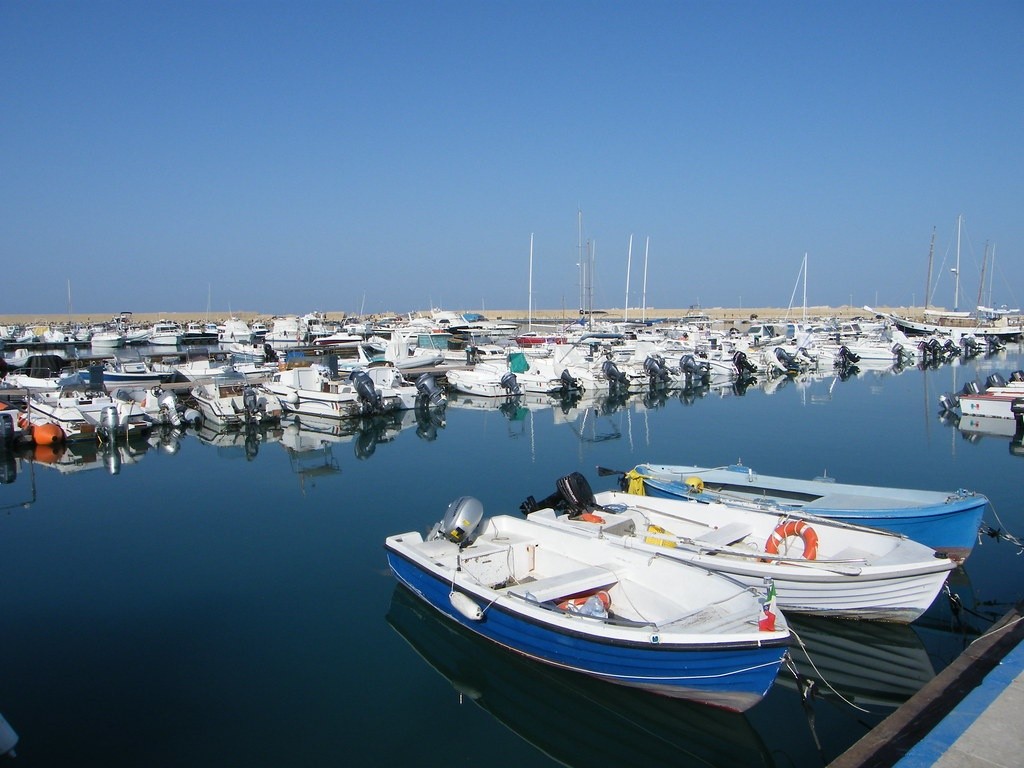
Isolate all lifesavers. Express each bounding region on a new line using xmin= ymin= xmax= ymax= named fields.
xmin=555 ymin=588 xmax=611 ymax=611
xmin=767 ymin=520 xmax=818 ymax=565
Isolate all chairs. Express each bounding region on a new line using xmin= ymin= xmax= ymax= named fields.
xmin=91 ymin=396 xmax=112 ymax=404
xmin=57 ymin=398 xmax=79 ymax=408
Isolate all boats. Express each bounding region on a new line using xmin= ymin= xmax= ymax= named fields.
xmin=937 ymin=408 xmax=1024 ymax=457
xmin=381 ymin=494 xmax=794 ymax=712
xmin=1 ymin=210 xmax=1024 ymax=460
xmin=593 ymin=456 xmax=990 ymax=569
xmin=775 ymin=611 xmax=937 ymax=706
xmin=907 ymin=565 xmax=993 ymax=635
xmin=515 ymin=467 xmax=965 ymax=628
xmin=383 ymin=581 xmax=772 ymax=766
xmin=0 ymin=388 xmax=675 ymax=484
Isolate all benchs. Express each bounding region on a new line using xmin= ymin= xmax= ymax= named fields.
xmin=497 ymin=560 xmax=630 ymax=603
xmin=677 ymin=520 xmax=753 ymax=556
